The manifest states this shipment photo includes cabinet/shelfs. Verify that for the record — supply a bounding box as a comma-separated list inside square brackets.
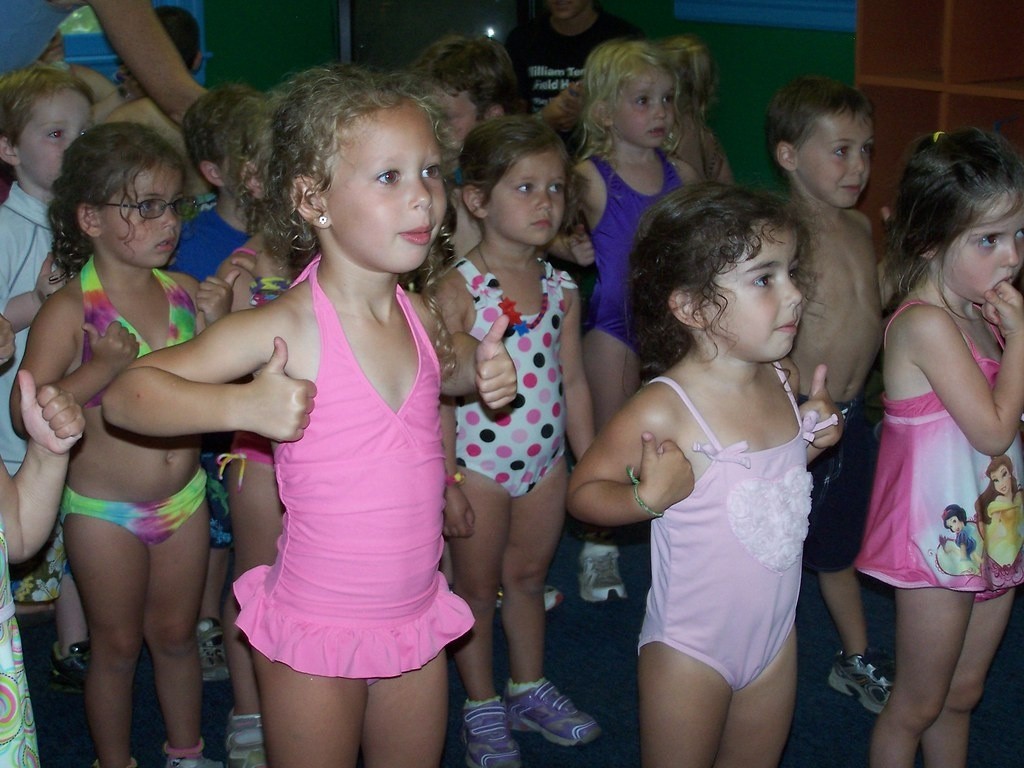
[856, 0, 1024, 263]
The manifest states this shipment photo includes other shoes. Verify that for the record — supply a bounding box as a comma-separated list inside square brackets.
[166, 756, 224, 768]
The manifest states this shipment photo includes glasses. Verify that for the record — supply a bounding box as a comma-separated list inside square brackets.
[85, 195, 195, 220]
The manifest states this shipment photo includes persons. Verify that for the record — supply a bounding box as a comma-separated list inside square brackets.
[1, 0, 1024, 768]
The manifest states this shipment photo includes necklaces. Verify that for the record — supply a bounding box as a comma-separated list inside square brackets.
[476, 242, 549, 336]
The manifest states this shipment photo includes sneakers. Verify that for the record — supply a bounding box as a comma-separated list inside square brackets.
[579, 531, 628, 602]
[502, 677, 601, 747]
[828, 648, 895, 714]
[496, 583, 564, 611]
[224, 705, 268, 768]
[460, 694, 521, 768]
[196, 617, 231, 682]
[38, 637, 92, 699]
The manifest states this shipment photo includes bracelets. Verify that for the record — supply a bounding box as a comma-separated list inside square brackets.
[625, 464, 664, 518]
[248, 292, 279, 307]
[249, 276, 292, 293]
[446, 472, 465, 489]
